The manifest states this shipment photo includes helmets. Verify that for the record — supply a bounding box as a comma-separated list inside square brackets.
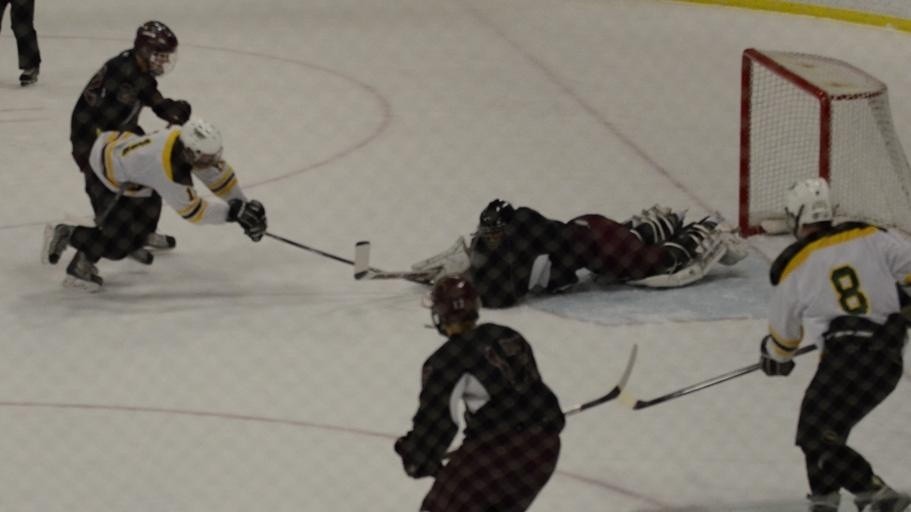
[784, 177, 833, 225]
[429, 272, 478, 335]
[478, 198, 516, 252]
[180, 117, 223, 167]
[137, 21, 178, 74]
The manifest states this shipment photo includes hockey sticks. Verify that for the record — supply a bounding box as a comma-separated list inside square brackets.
[441, 344, 639, 460]
[354, 241, 439, 279]
[615, 341, 818, 410]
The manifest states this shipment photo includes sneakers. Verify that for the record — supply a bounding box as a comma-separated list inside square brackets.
[143, 232, 176, 248]
[49, 224, 78, 264]
[131, 247, 154, 265]
[856, 474, 911, 512]
[66, 249, 103, 287]
[19, 63, 39, 80]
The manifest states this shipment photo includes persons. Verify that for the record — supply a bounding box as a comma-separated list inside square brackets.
[0, 1, 43, 87]
[70, 20, 192, 266]
[41, 118, 267, 294]
[394, 278, 565, 512]
[427, 198, 749, 311]
[757, 176, 910, 512]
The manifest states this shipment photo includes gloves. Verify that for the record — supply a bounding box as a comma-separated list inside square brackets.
[225, 198, 268, 242]
[761, 334, 795, 376]
[154, 99, 192, 125]
[394, 429, 444, 477]
